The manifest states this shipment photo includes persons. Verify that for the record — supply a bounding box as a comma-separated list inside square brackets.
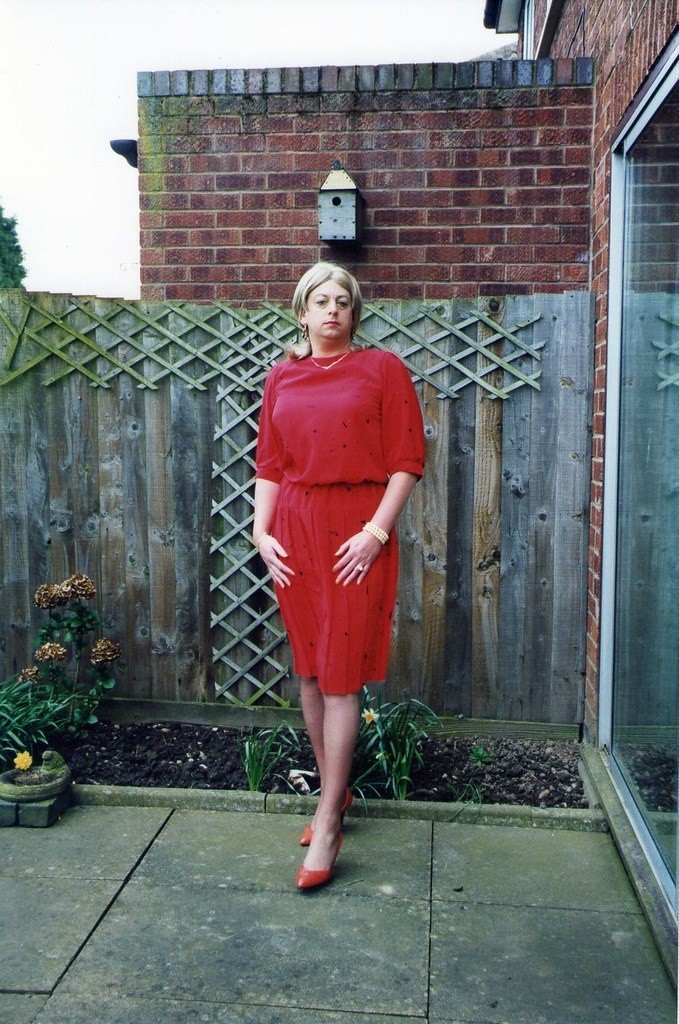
[250, 260, 425, 890]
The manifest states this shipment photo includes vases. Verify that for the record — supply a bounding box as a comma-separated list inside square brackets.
[0, 750, 71, 802]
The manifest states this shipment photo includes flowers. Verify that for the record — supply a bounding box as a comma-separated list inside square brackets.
[14, 750, 32, 770]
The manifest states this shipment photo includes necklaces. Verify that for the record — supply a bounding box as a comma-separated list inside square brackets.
[311, 349, 350, 369]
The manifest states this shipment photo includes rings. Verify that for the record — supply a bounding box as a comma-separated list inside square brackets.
[357, 564, 363, 571]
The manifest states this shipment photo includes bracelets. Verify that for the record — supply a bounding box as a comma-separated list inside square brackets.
[363, 522, 389, 545]
[256, 534, 265, 548]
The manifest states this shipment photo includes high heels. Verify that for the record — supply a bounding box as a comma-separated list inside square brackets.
[298, 784, 353, 846]
[295, 830, 343, 890]
